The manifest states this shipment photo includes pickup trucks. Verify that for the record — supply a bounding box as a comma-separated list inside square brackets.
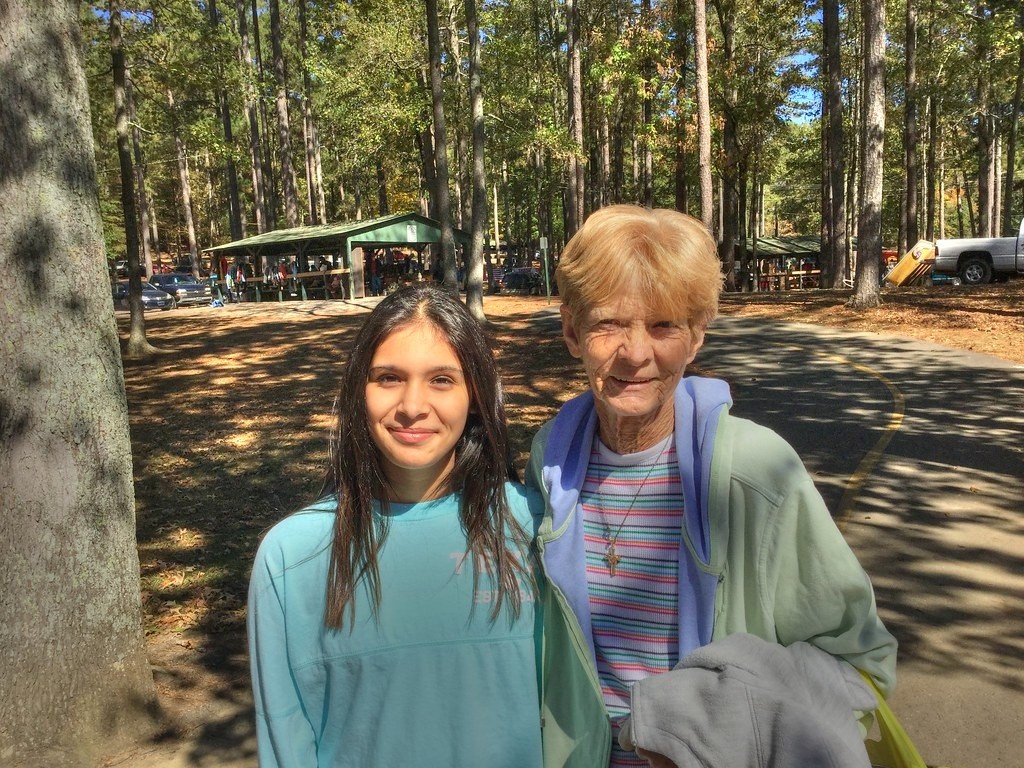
[934, 216, 1024, 286]
[149, 273, 214, 307]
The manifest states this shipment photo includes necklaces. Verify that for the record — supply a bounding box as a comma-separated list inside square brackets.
[597, 418, 676, 576]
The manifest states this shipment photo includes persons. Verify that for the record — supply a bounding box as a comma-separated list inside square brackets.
[365, 253, 386, 296]
[277, 257, 331, 280]
[247, 282, 545, 768]
[525, 204, 897, 768]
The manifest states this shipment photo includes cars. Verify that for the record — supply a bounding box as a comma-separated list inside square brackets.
[114, 259, 129, 276]
[112, 279, 173, 313]
